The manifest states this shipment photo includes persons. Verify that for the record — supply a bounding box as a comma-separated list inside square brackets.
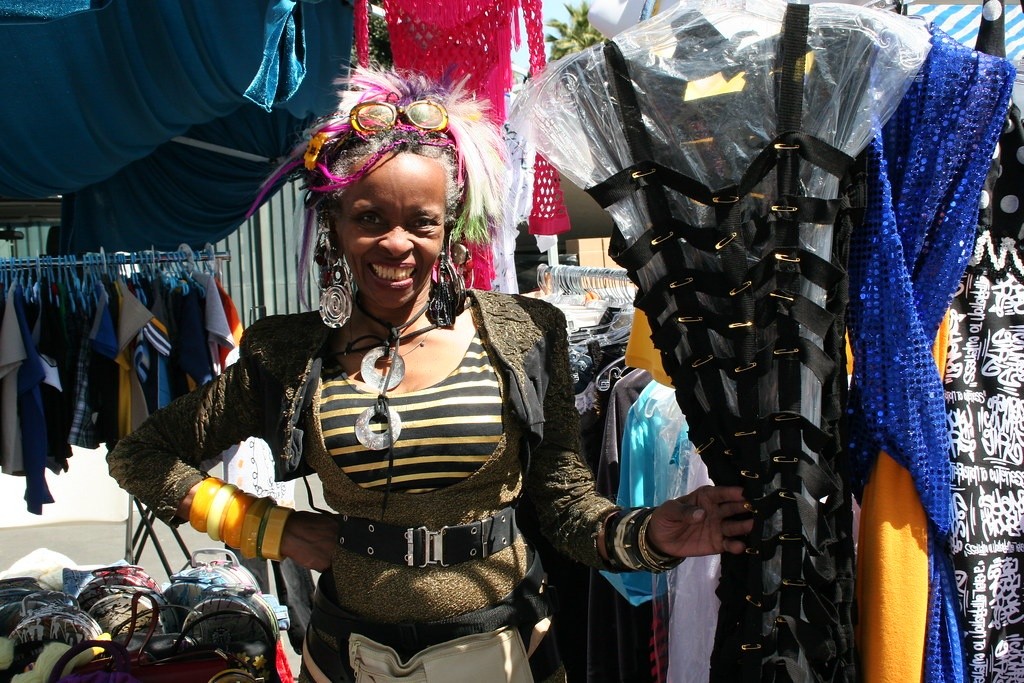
[106, 62, 756, 683]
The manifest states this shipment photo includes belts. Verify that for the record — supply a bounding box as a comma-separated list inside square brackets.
[334, 505, 520, 569]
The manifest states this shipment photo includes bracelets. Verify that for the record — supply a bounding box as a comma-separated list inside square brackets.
[190, 477, 295, 562]
[604, 506, 686, 574]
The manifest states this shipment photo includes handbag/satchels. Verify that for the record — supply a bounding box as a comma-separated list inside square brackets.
[0, 559, 276, 683]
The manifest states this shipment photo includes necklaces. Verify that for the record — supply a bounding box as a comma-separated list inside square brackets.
[352, 300, 434, 451]
[349, 306, 436, 362]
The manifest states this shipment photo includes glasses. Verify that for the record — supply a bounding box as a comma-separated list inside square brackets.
[350, 100, 447, 132]
